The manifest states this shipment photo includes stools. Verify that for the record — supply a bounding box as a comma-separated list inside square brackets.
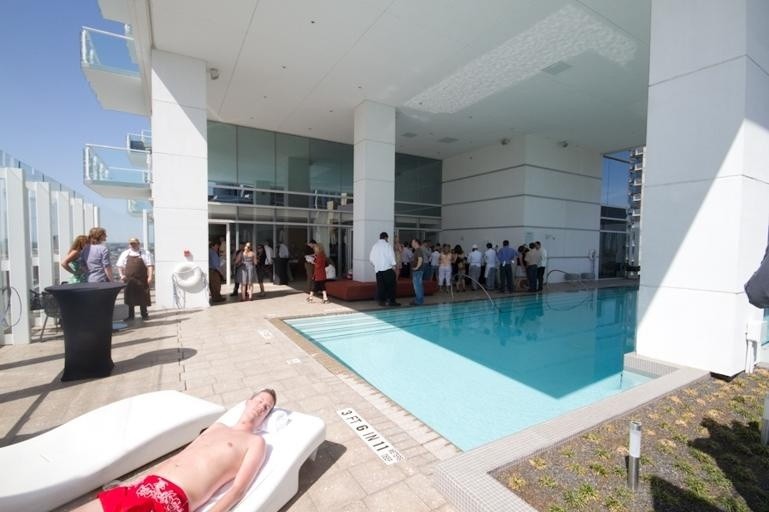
[565, 273, 580, 287]
[581, 272, 595, 285]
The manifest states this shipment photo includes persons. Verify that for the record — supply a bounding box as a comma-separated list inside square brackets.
[61, 235, 88, 284]
[66, 389, 276, 512]
[116, 238, 154, 322]
[81, 228, 113, 283]
[394, 234, 547, 305]
[369, 232, 401, 307]
[209, 235, 336, 304]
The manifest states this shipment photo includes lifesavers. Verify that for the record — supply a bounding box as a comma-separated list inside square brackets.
[174, 263, 202, 289]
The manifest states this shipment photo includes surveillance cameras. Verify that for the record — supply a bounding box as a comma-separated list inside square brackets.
[502, 139, 510, 145]
[562, 142, 568, 148]
[211, 71, 219, 80]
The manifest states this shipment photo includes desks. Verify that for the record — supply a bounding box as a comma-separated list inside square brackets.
[47, 282, 131, 384]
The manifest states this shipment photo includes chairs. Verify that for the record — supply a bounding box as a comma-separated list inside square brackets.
[38, 292, 65, 343]
[0, 389, 231, 512]
[128, 396, 326, 511]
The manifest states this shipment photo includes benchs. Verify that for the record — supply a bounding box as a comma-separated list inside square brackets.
[324, 277, 435, 305]
